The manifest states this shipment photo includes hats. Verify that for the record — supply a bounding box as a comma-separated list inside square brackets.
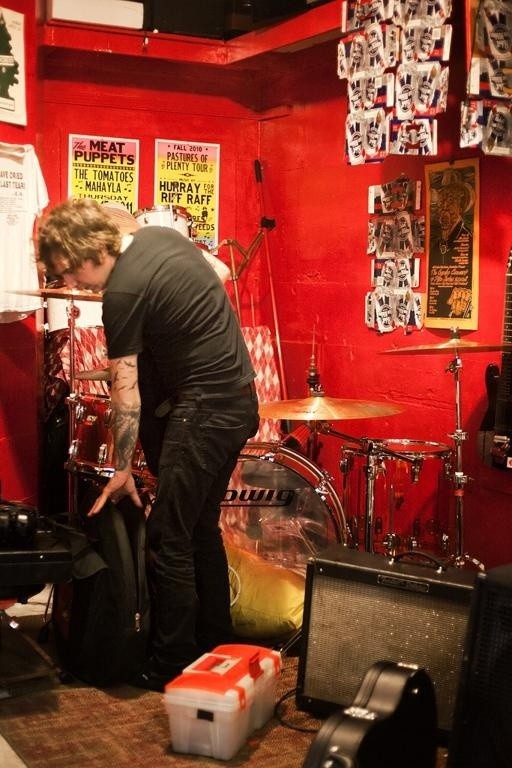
[434, 169, 476, 212]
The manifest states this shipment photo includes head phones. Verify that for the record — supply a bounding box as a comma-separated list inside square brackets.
[0, 498, 41, 546]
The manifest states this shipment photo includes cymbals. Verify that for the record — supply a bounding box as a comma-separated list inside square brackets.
[6, 288, 104, 302]
[69, 366, 109, 380]
[378, 339, 510, 353]
[257, 396, 403, 421]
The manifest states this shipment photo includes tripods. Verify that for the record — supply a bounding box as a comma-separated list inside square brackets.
[432, 359, 487, 574]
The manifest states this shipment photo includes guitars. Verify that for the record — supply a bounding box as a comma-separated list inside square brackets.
[478, 247, 512, 470]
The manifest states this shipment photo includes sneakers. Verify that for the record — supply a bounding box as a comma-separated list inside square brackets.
[138, 661, 181, 686]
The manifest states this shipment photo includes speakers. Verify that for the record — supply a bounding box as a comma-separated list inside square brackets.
[445, 562, 511, 767]
[295, 545, 474, 741]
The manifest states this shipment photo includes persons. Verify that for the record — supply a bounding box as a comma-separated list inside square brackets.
[38, 199, 259, 692]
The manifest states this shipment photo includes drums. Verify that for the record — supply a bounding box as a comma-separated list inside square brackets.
[135, 205, 193, 241]
[340, 439, 451, 564]
[62, 391, 158, 487]
[216, 442, 359, 579]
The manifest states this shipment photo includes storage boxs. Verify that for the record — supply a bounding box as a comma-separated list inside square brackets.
[161, 642, 284, 763]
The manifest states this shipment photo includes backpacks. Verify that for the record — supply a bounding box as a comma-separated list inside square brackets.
[69, 505, 152, 687]
